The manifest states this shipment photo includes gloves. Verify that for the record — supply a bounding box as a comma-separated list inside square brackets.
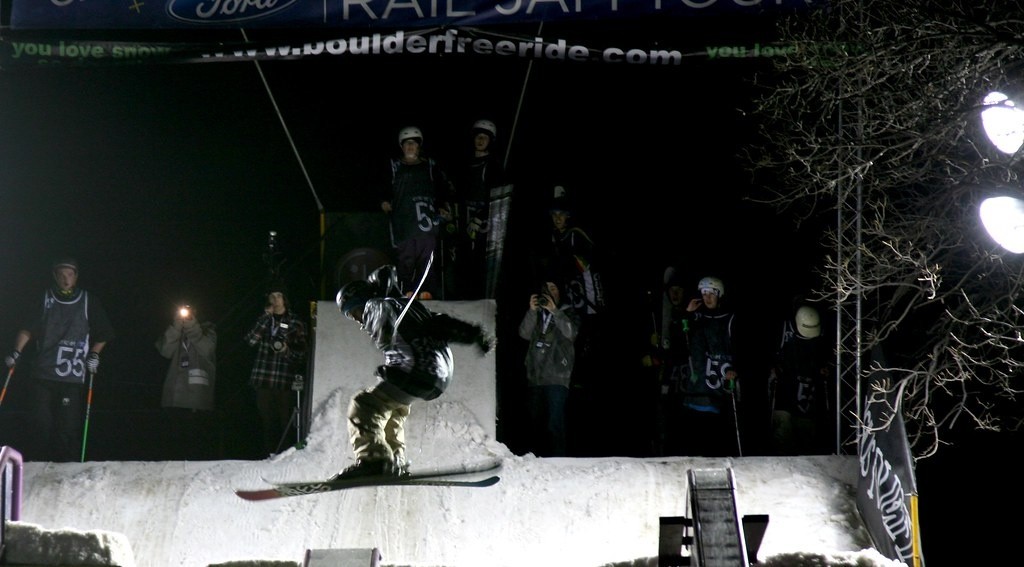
[474, 324, 492, 354]
[4, 349, 22, 368]
[84, 351, 100, 374]
[385, 349, 414, 372]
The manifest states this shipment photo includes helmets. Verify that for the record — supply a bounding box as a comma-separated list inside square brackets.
[398, 126, 424, 149]
[665, 272, 691, 299]
[796, 307, 821, 337]
[336, 280, 374, 317]
[548, 201, 571, 218]
[698, 277, 725, 297]
[50, 257, 82, 277]
[472, 119, 498, 141]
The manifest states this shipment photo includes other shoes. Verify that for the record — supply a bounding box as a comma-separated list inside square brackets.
[406, 291, 419, 299]
[325, 453, 411, 480]
[418, 291, 433, 300]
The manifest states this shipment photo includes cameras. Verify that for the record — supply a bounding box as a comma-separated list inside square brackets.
[268, 323, 288, 352]
[536, 295, 548, 306]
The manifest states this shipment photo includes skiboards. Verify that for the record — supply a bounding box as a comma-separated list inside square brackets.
[235, 460, 505, 502]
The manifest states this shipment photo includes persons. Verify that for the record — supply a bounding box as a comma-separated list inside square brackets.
[328, 280, 494, 478]
[444, 120, 507, 301]
[157, 299, 218, 460]
[371, 127, 458, 301]
[518, 194, 831, 459]
[243, 288, 307, 463]
[5, 256, 115, 466]
